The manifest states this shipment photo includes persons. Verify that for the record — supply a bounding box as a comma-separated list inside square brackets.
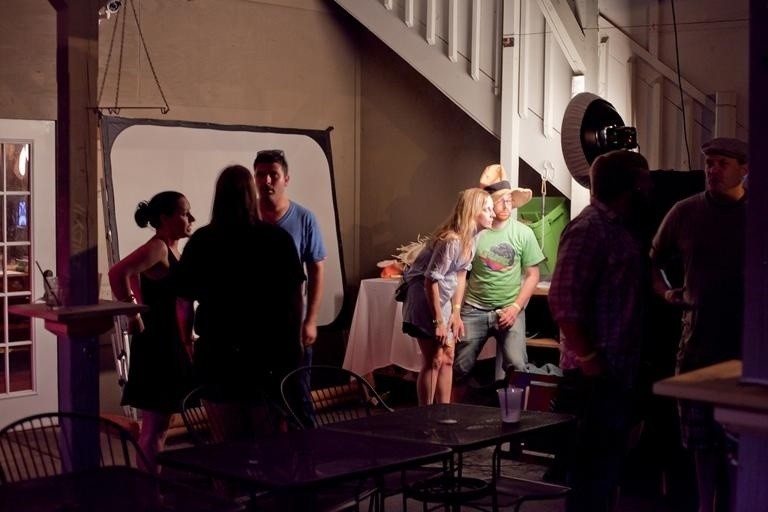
[649, 136, 750, 512]
[395, 188, 497, 405]
[252, 150, 327, 432]
[539, 149, 653, 487]
[108, 190, 196, 473]
[451, 163, 546, 404]
[177, 165, 309, 444]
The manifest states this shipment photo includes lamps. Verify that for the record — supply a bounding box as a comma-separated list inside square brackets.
[559, 92, 639, 193]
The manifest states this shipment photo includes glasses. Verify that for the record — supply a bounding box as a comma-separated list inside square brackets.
[256, 148, 286, 167]
[496, 196, 515, 205]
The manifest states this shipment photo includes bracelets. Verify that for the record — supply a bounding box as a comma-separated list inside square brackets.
[120, 294, 136, 303]
[511, 302, 522, 312]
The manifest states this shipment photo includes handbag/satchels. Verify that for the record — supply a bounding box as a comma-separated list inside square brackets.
[393, 273, 426, 304]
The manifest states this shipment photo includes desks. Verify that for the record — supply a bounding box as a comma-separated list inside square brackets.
[1, 464, 240, 512]
[322, 402, 574, 512]
[156, 431, 454, 512]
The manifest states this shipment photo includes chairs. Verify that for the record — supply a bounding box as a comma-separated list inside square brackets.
[180, 377, 379, 512]
[1, 414, 152, 483]
[280, 365, 452, 512]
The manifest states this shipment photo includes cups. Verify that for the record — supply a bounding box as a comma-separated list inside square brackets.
[497, 388, 523, 429]
[43, 277, 67, 311]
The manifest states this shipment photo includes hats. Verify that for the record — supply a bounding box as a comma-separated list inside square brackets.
[479, 161, 532, 213]
[700, 137, 749, 164]
